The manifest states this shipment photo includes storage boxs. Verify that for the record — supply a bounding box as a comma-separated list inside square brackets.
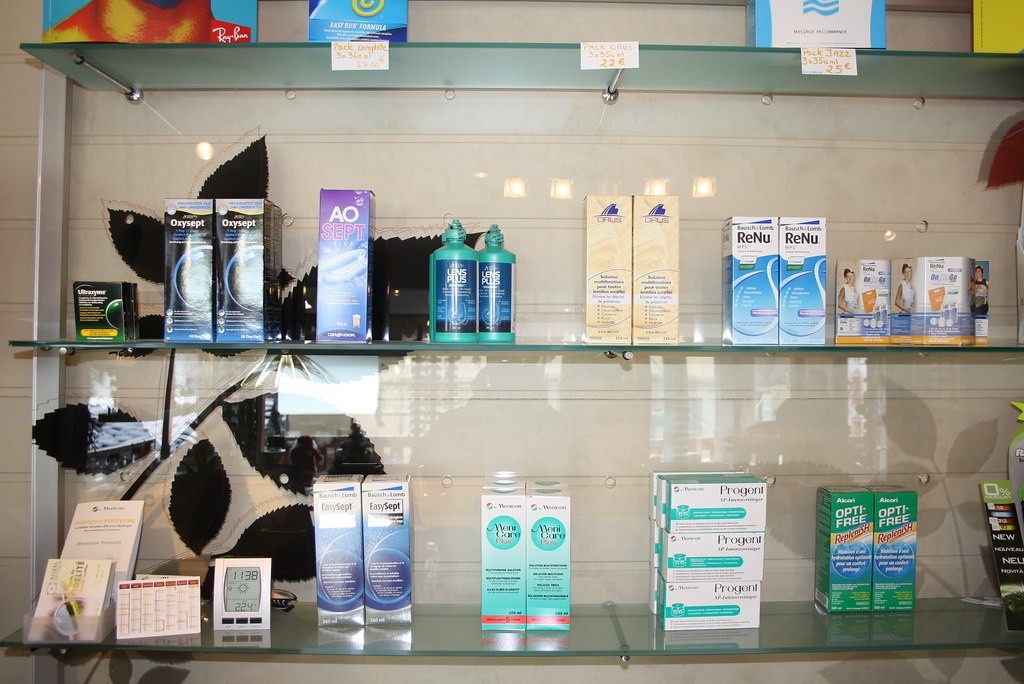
[583, 196, 680, 345]
[744, 0, 885, 49]
[482, 480, 572, 633]
[649, 469, 767, 631]
[74, 282, 140, 342]
[815, 484, 917, 613]
[720, 216, 826, 346]
[166, 197, 284, 345]
[316, 187, 375, 343]
[313, 473, 413, 627]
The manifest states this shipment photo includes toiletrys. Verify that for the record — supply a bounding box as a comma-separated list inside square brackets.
[425, 217, 481, 348]
[478, 222, 519, 345]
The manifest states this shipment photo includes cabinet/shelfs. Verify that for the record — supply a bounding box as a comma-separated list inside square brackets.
[19, 42, 1024, 684]
